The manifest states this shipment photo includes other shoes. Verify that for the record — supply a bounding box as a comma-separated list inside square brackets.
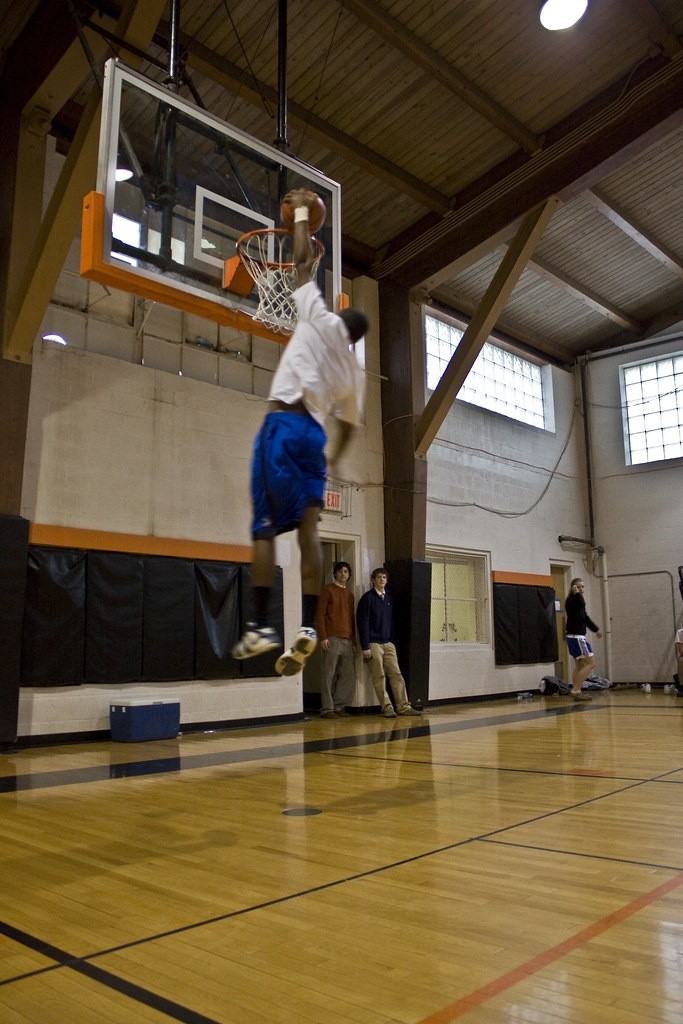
[640, 683, 651, 693]
[401, 708, 422, 716]
[325, 711, 339, 719]
[677, 687, 683, 697]
[573, 692, 591, 701]
[336, 709, 349, 718]
[384, 708, 396, 718]
[663, 684, 674, 694]
[569, 691, 587, 700]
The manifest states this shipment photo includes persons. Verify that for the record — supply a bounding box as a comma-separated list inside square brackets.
[675, 620, 683, 697]
[317, 559, 358, 719]
[562, 578, 603, 701]
[231, 189, 372, 677]
[356, 568, 420, 718]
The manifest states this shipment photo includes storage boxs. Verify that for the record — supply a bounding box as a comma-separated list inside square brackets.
[109, 696, 180, 742]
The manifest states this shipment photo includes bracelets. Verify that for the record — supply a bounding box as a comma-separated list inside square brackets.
[293, 204, 310, 224]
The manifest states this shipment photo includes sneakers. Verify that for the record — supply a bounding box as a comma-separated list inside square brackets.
[231, 621, 281, 660]
[276, 627, 316, 676]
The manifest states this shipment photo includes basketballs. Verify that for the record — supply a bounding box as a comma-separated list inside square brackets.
[280, 188, 327, 235]
